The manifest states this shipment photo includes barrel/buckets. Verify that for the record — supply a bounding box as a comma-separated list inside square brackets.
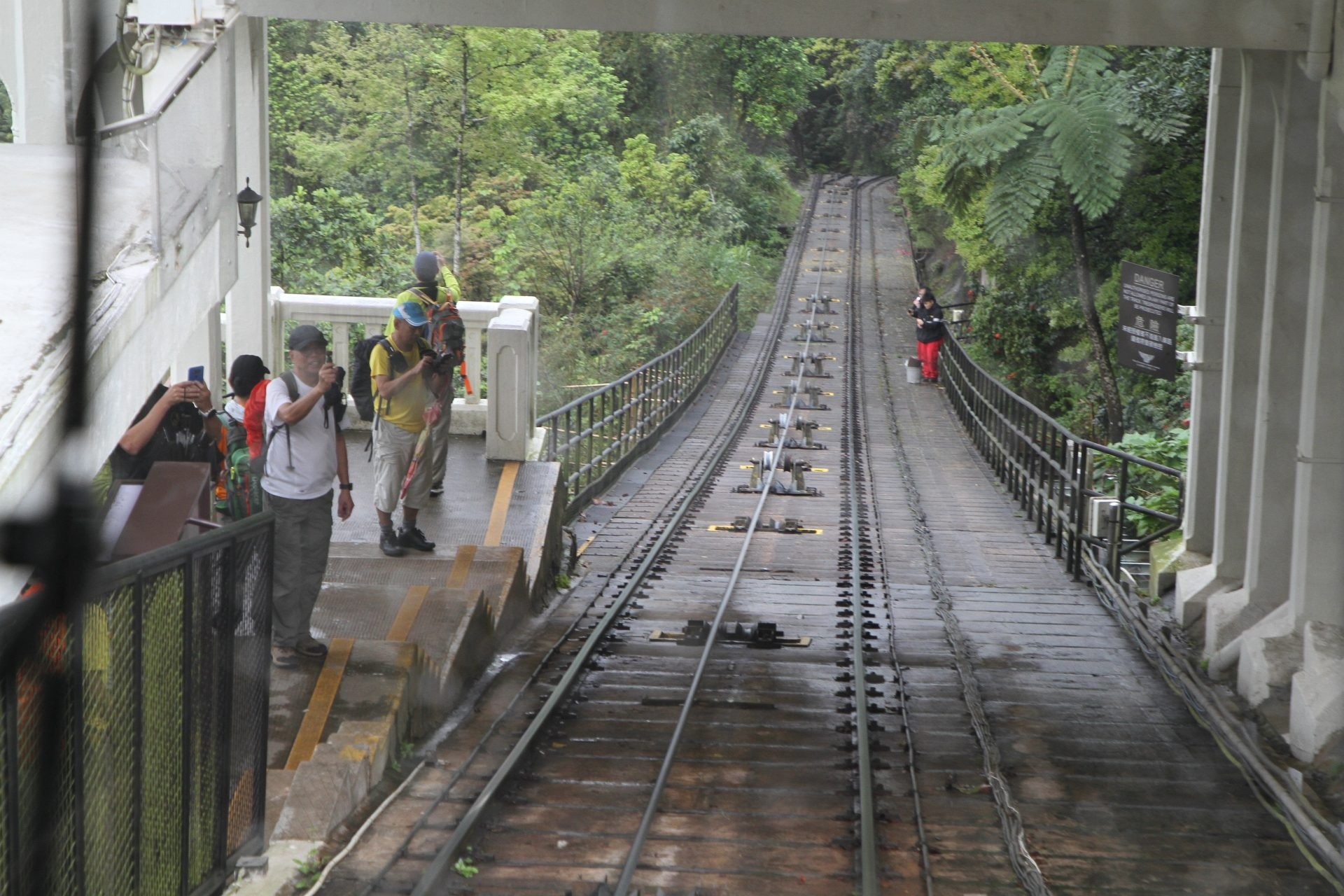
[906, 366, 922, 383]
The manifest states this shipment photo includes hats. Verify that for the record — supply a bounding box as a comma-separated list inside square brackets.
[289, 324, 328, 351]
[918, 292, 933, 303]
[230, 355, 271, 377]
[394, 302, 432, 326]
[414, 251, 438, 276]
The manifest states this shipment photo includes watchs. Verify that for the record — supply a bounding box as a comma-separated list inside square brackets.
[197, 408, 215, 420]
[340, 482, 353, 491]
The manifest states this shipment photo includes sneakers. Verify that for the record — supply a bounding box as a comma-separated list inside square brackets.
[380, 530, 408, 557]
[398, 527, 436, 550]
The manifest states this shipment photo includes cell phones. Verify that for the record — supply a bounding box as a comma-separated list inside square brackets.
[187, 366, 204, 381]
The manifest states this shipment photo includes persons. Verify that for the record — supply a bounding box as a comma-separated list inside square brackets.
[908, 286, 944, 384]
[384, 249, 461, 496]
[213, 353, 273, 637]
[259, 324, 356, 669]
[109, 378, 225, 482]
[369, 302, 452, 556]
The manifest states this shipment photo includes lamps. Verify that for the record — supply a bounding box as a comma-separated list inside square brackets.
[237, 177, 263, 247]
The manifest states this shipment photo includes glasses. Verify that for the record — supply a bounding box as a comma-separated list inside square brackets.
[296, 344, 325, 358]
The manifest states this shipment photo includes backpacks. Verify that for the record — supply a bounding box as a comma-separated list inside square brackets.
[213, 410, 265, 520]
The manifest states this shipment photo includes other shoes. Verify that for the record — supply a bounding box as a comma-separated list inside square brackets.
[270, 645, 299, 667]
[295, 636, 328, 655]
[211, 612, 242, 629]
[430, 485, 444, 496]
[254, 621, 272, 638]
[923, 378, 937, 385]
